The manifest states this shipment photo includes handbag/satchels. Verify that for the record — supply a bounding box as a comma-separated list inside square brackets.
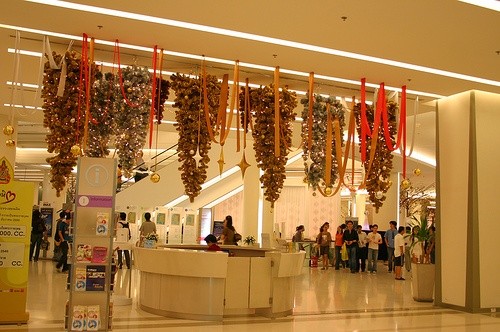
[233, 233, 241, 242]
[328, 248, 333, 259]
[342, 243, 348, 260]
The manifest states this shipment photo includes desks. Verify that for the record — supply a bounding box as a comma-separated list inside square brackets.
[296, 241, 335, 266]
[112, 241, 135, 305]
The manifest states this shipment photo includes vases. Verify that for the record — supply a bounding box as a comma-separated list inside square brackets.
[148, 239, 157, 246]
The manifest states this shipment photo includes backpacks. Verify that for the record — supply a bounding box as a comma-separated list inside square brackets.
[118, 222, 131, 240]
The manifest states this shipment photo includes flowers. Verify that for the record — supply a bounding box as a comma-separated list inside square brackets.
[146, 230, 159, 241]
[244, 235, 256, 246]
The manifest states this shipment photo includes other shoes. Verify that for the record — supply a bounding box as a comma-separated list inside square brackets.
[320, 268, 325, 270]
[361, 269, 365, 272]
[395, 277, 406, 280]
[119, 263, 123, 269]
[367, 271, 377, 274]
[56, 268, 62, 273]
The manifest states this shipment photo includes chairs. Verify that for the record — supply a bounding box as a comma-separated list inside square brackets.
[279, 238, 346, 268]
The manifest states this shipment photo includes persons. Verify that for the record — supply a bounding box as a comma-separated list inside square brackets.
[53, 211, 71, 274]
[139, 212, 157, 242]
[29, 210, 46, 263]
[115, 212, 131, 270]
[316, 221, 382, 274]
[204, 215, 236, 251]
[293, 225, 310, 250]
[384, 221, 435, 280]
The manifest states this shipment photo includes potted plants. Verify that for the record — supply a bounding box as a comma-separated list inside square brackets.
[403, 213, 436, 303]
